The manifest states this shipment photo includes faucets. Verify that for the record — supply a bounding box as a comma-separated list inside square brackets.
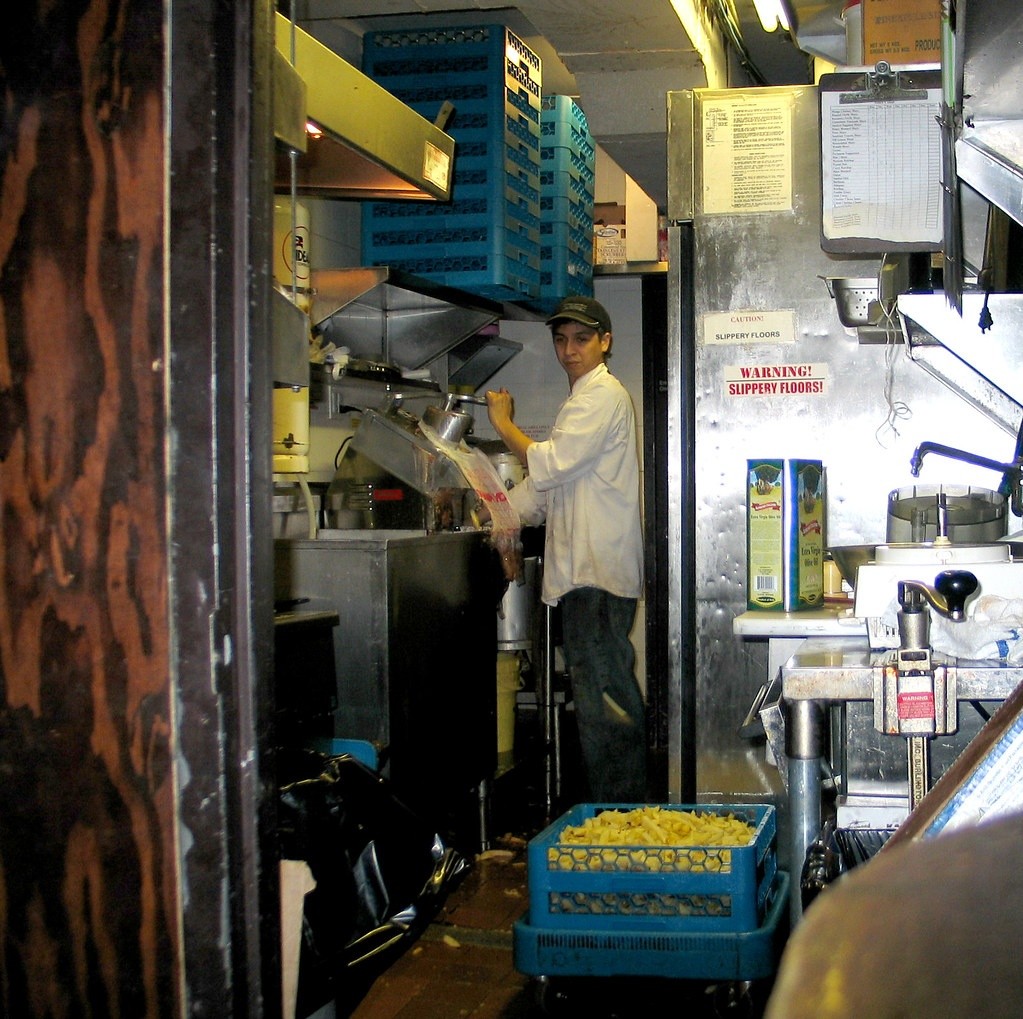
[910, 441, 1021, 479]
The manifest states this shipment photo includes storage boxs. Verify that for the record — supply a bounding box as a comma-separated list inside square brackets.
[357, 20, 597, 310]
[513, 797, 790, 981]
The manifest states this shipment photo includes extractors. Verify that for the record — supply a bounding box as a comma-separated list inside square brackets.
[311, 267, 503, 370]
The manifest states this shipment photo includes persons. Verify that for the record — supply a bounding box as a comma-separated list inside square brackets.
[487, 298, 647, 801]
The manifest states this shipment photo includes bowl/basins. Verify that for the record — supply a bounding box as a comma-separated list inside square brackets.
[825, 545, 875, 589]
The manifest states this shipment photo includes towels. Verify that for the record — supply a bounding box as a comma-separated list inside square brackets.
[880, 593, 1022, 659]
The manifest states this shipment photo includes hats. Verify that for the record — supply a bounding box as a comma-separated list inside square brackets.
[545, 296, 611, 331]
